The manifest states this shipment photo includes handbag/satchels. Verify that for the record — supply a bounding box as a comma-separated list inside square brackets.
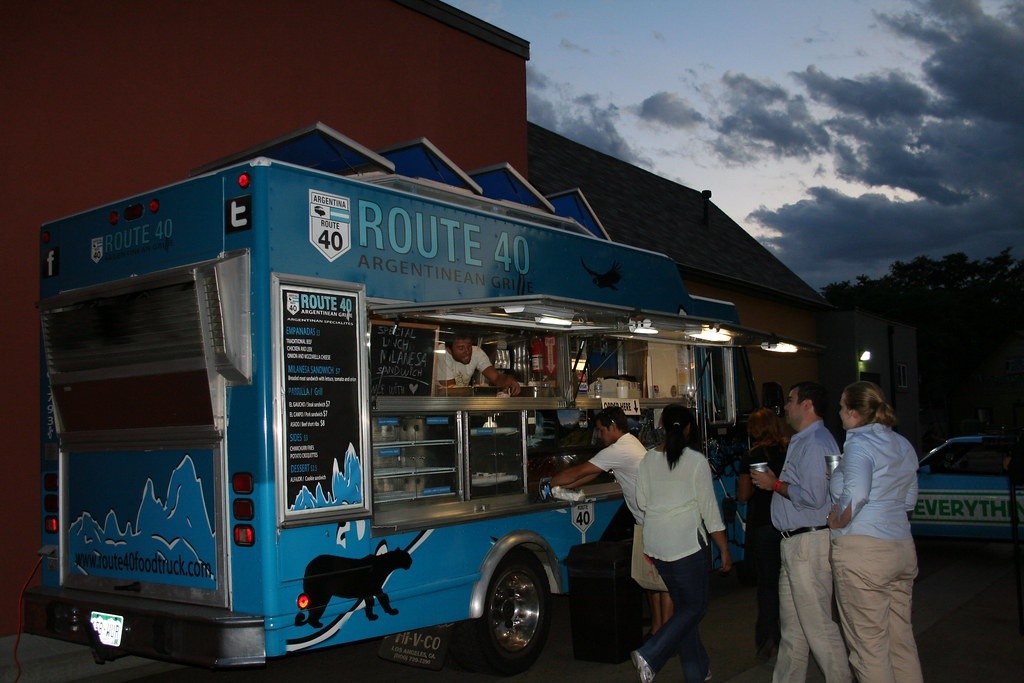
[629, 521, 670, 593]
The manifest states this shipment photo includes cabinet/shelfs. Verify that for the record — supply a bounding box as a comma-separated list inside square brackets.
[371, 408, 528, 505]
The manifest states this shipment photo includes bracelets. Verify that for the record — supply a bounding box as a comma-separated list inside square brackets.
[773, 479, 782, 491]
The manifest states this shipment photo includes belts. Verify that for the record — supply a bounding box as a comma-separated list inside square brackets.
[781, 524, 829, 540]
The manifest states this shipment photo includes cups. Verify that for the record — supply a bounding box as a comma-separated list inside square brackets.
[617, 380, 641, 399]
[825, 455, 841, 479]
[749, 463, 768, 473]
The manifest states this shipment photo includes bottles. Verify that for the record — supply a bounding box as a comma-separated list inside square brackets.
[594, 378, 603, 398]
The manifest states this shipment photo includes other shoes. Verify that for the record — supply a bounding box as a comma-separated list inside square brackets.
[630, 648, 658, 683]
[704, 668, 713, 681]
[756, 634, 775, 663]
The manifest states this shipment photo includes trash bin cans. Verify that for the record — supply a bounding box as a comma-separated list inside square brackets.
[560, 540, 643, 663]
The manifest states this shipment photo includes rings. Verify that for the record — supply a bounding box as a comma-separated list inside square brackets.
[754, 481, 757, 484]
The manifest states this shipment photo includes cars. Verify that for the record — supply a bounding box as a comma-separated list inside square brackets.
[906, 433, 1024, 553]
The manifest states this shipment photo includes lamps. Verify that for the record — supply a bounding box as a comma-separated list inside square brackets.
[762, 341, 798, 354]
[683, 326, 732, 342]
[507, 305, 575, 326]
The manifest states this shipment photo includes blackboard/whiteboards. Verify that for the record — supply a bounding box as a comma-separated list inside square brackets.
[367, 319, 439, 397]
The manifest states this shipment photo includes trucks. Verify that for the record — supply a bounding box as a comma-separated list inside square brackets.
[19, 158, 788, 678]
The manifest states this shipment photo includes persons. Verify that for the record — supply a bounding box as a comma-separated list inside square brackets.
[630, 404, 733, 683]
[550, 406, 673, 638]
[750, 381, 855, 683]
[738, 408, 788, 667]
[434, 326, 521, 396]
[827, 381, 923, 683]
